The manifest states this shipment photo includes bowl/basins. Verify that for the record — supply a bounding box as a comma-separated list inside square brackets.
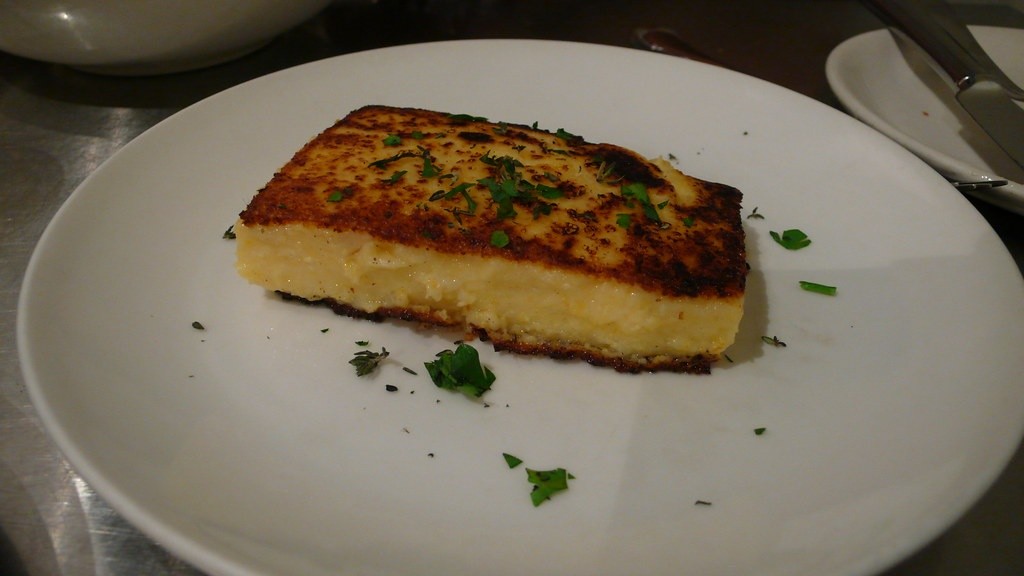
[0, 0, 336, 76]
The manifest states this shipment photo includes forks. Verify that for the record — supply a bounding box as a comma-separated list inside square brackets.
[636, 29, 1008, 192]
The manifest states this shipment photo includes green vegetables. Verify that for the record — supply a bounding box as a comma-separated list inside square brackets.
[327, 117, 838, 502]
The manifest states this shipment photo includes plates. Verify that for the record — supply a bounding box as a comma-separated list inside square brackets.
[18, 39, 1024, 576]
[825, 22, 1024, 211]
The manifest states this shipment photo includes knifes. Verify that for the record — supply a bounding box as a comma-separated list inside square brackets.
[866, 0, 1024, 170]
